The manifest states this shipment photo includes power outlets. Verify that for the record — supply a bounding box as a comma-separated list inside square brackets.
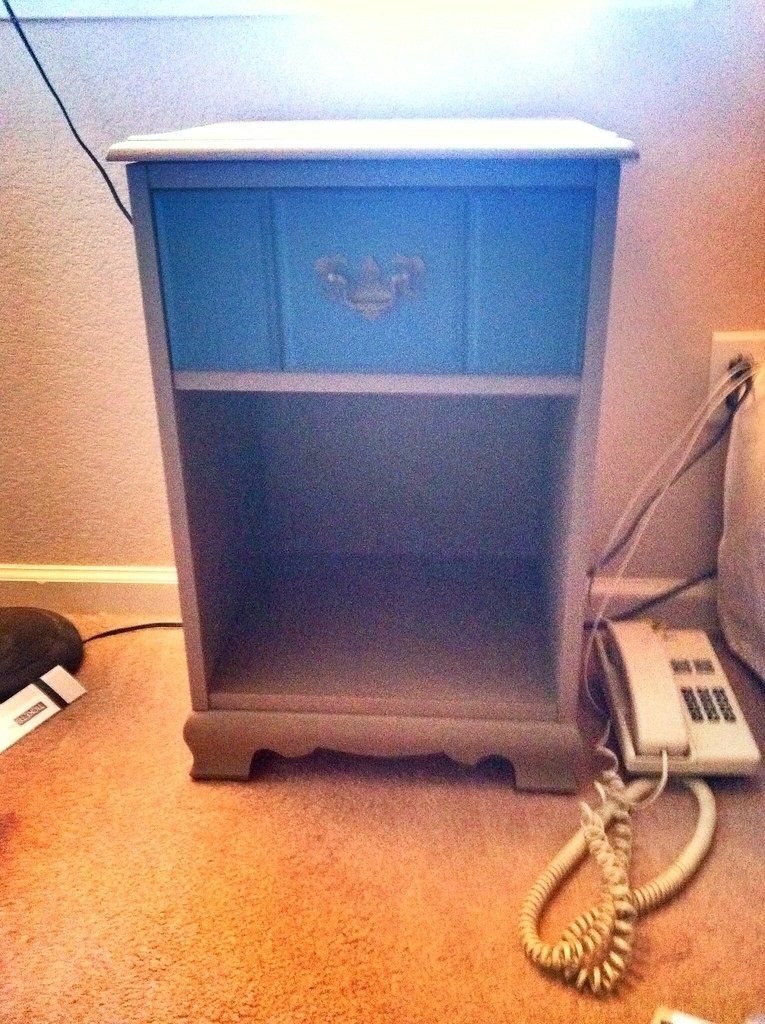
[705, 330, 765, 422]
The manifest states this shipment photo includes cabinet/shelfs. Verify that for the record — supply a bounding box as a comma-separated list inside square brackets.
[105, 120, 643, 800]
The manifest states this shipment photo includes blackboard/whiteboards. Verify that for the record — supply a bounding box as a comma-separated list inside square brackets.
[593, 622, 761, 775]
